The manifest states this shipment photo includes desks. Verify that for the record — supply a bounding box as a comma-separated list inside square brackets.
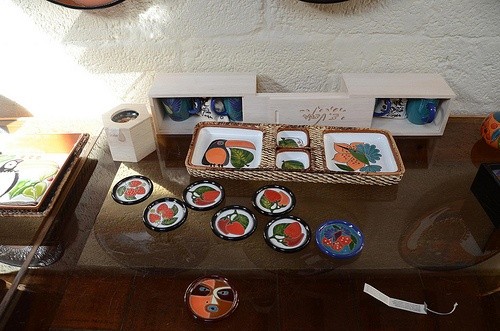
[0, 116, 500, 330]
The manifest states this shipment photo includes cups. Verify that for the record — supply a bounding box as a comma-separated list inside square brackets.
[163, 98, 200, 122]
[211, 97, 243, 122]
[406, 98, 441, 125]
